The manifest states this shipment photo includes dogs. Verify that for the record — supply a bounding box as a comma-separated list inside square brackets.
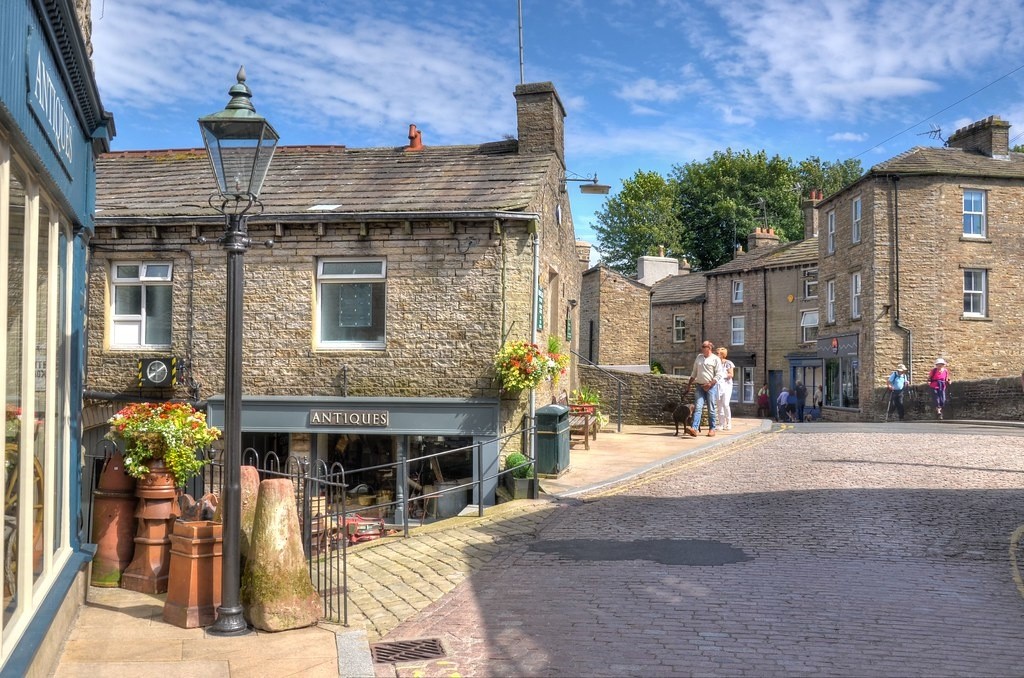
[672, 403, 701, 436]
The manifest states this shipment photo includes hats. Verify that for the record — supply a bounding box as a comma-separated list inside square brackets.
[896, 364, 908, 372]
[934, 359, 946, 365]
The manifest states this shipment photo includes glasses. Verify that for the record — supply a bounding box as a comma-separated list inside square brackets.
[702, 346, 709, 348]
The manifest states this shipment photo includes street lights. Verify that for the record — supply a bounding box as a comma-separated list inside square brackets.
[195, 65, 280, 639]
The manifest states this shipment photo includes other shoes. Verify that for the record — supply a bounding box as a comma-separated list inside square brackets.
[726, 423, 731, 430]
[899, 417, 905, 421]
[718, 425, 724, 431]
[938, 413, 943, 419]
[708, 429, 715, 436]
[686, 428, 698, 437]
[888, 413, 897, 419]
[937, 408, 941, 415]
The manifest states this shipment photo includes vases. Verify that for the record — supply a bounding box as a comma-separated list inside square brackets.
[130, 430, 169, 461]
[500, 391, 519, 399]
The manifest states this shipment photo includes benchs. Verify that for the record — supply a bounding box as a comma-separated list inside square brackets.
[552, 389, 604, 450]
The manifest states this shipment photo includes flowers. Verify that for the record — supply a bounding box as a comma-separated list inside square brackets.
[103, 400, 222, 489]
[493, 339, 550, 399]
[543, 332, 571, 389]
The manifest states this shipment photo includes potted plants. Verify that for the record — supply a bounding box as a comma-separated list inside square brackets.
[566, 385, 608, 435]
[504, 451, 540, 499]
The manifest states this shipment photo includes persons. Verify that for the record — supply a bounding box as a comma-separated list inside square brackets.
[927, 359, 952, 420]
[685, 340, 722, 437]
[776, 380, 823, 423]
[757, 383, 771, 418]
[714, 346, 735, 431]
[886, 363, 910, 421]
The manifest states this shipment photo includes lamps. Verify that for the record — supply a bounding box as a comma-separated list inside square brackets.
[559, 170, 611, 194]
[196, 64, 281, 216]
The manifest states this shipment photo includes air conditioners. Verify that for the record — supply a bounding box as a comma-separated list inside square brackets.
[139, 356, 183, 388]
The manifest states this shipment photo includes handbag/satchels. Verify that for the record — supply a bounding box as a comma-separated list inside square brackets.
[818, 401, 822, 406]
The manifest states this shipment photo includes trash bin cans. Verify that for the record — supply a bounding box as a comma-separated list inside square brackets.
[535, 404, 570, 473]
